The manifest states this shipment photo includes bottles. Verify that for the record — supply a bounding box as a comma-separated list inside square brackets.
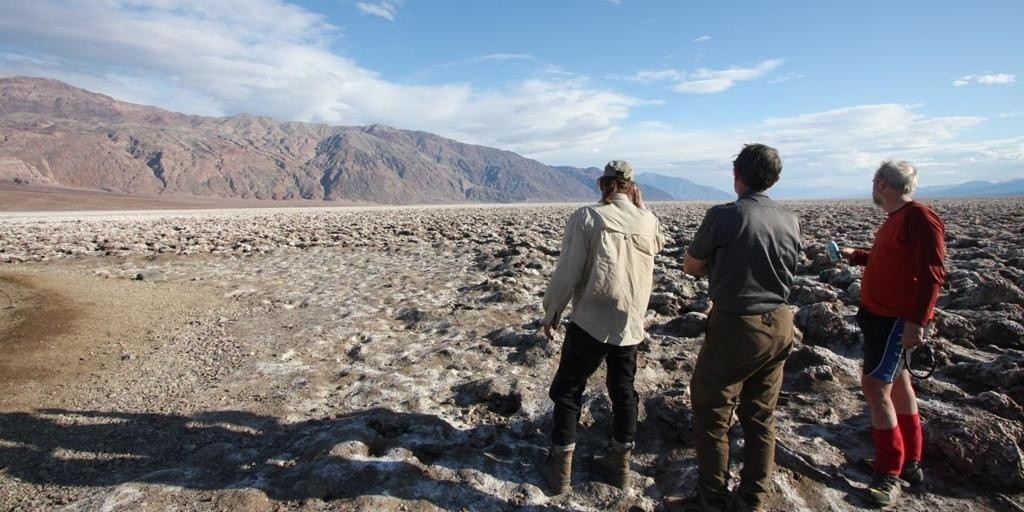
[825, 236, 842, 264]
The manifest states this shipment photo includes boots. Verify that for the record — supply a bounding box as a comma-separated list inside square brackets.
[536, 442, 576, 494]
[581, 437, 635, 488]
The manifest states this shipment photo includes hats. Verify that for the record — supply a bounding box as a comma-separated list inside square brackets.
[604, 160, 634, 182]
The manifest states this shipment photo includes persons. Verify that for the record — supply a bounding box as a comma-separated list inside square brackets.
[825, 160, 946, 508]
[534, 158, 665, 495]
[662, 143, 802, 511]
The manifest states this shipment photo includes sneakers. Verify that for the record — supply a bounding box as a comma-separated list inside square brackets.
[864, 468, 923, 504]
[663, 485, 762, 512]
